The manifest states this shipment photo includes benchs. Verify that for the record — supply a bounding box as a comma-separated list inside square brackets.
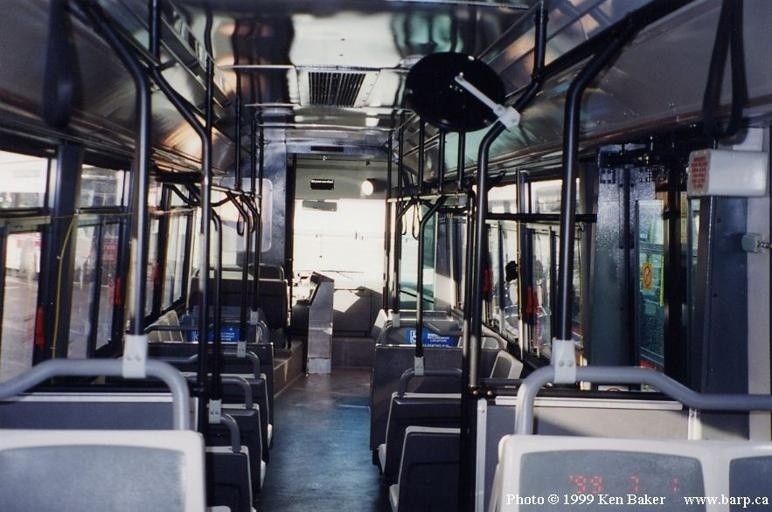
[0, 262, 291, 512]
[367, 317, 772, 511]
